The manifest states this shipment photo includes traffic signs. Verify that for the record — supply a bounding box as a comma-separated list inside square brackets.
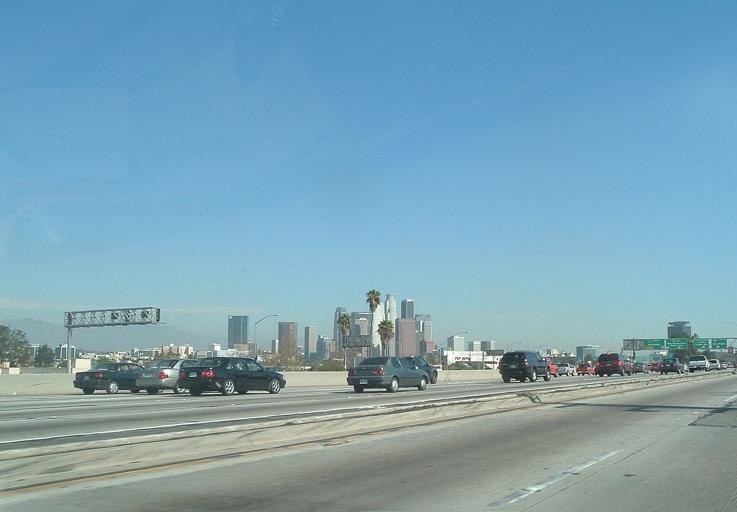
[643, 339, 665, 349]
[666, 338, 688, 350]
[712, 338, 728, 349]
[693, 338, 709, 349]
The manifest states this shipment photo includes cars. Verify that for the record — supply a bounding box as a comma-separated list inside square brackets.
[496, 350, 734, 382]
[346, 355, 439, 392]
[72, 356, 287, 396]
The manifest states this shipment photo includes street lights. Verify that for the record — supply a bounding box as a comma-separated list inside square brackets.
[255, 313, 279, 363]
